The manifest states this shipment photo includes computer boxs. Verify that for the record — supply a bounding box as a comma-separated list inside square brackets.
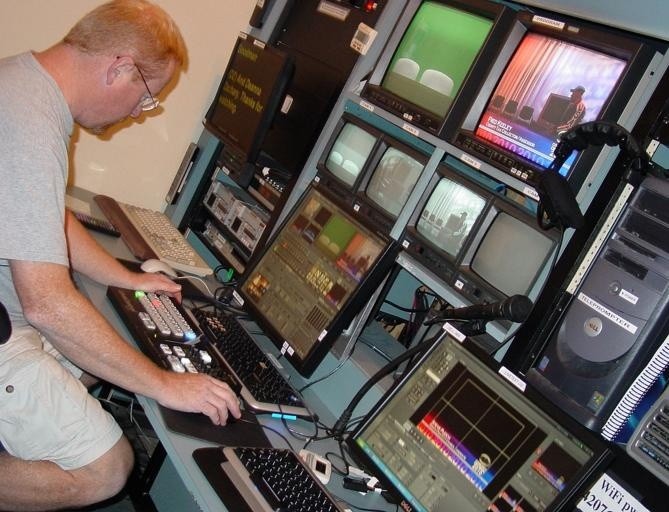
[522, 171, 669, 434]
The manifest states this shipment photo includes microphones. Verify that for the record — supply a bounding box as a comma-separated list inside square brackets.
[431, 294, 534, 324]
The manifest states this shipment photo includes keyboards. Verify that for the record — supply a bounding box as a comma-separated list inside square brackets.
[186, 307, 317, 420]
[219, 445, 344, 511]
[120, 201, 215, 278]
[105, 284, 243, 398]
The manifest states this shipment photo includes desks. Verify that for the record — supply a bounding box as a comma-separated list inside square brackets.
[58, 183, 408, 512]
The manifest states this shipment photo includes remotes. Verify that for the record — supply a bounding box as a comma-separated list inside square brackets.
[72, 212, 121, 236]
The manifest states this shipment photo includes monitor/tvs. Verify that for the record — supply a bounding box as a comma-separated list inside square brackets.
[204, 32, 292, 168]
[355, 135, 431, 223]
[407, 165, 495, 270]
[459, 198, 564, 299]
[235, 182, 403, 378]
[316, 111, 385, 195]
[450, 10, 650, 205]
[360, 0, 514, 142]
[347, 323, 615, 511]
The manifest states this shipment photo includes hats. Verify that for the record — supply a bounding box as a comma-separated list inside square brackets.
[570, 85, 585, 92]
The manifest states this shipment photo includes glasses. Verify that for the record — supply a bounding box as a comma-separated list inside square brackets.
[117, 56, 160, 112]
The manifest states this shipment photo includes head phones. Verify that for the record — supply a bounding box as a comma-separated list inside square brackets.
[531, 117, 649, 233]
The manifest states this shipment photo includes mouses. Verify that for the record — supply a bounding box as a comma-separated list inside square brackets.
[140, 258, 178, 281]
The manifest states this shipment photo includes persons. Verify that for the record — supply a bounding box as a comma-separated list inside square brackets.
[1, 1, 241, 511]
[542, 86, 586, 138]
